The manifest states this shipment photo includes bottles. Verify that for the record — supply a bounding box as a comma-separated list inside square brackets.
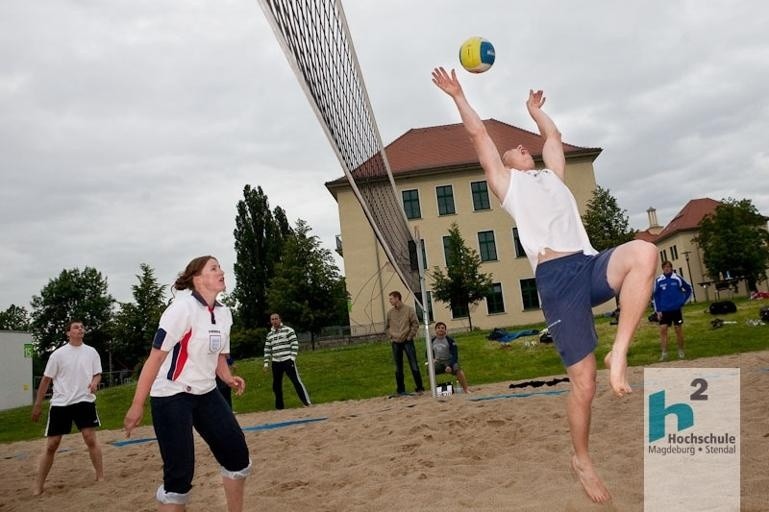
[455, 380, 462, 394]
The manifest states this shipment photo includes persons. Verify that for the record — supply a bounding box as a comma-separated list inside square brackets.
[123, 255, 253, 512]
[424, 322, 472, 394]
[650, 261, 694, 362]
[30, 319, 104, 497]
[384, 291, 425, 399]
[263, 313, 311, 410]
[429, 63, 659, 506]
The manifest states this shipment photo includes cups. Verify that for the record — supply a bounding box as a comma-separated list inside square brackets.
[446, 384, 452, 395]
[436, 385, 442, 398]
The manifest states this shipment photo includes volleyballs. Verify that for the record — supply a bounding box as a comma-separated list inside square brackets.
[459, 36, 495, 72]
